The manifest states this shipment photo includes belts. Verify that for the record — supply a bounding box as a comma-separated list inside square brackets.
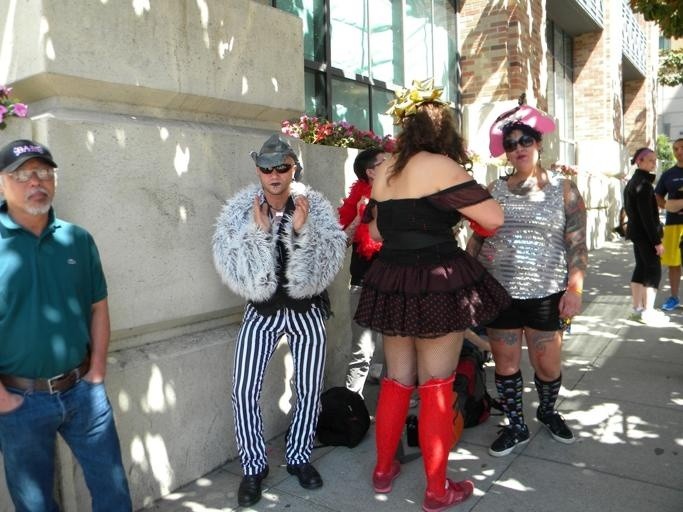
[1, 359, 93, 394]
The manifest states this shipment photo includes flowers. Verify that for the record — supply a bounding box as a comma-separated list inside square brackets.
[385, 78, 444, 125]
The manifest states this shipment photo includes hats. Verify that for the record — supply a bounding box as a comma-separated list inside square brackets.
[0, 140, 57, 173]
[490, 105, 557, 158]
[250, 134, 299, 169]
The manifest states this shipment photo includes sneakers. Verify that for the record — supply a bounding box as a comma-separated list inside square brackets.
[373, 459, 401, 493]
[630, 308, 671, 324]
[662, 296, 681, 311]
[421, 477, 474, 512]
[536, 405, 576, 444]
[489, 424, 530, 457]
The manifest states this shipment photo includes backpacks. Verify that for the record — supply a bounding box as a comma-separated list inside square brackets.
[315, 387, 371, 449]
[453, 338, 504, 428]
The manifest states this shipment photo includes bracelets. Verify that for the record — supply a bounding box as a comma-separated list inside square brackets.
[470, 223, 496, 238]
[569, 288, 586, 295]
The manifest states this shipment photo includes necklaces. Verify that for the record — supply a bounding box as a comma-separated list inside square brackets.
[267, 202, 285, 217]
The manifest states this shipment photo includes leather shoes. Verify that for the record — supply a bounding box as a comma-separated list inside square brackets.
[238, 465, 269, 507]
[287, 463, 323, 489]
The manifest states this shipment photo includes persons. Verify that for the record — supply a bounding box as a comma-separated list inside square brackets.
[212, 132, 350, 506]
[654, 138, 683, 311]
[466, 92, 589, 458]
[623, 147, 671, 322]
[337, 147, 392, 399]
[352, 80, 504, 512]
[0, 139, 134, 511]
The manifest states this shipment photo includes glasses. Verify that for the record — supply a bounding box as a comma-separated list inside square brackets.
[259, 164, 291, 174]
[504, 134, 535, 152]
[8, 167, 54, 182]
